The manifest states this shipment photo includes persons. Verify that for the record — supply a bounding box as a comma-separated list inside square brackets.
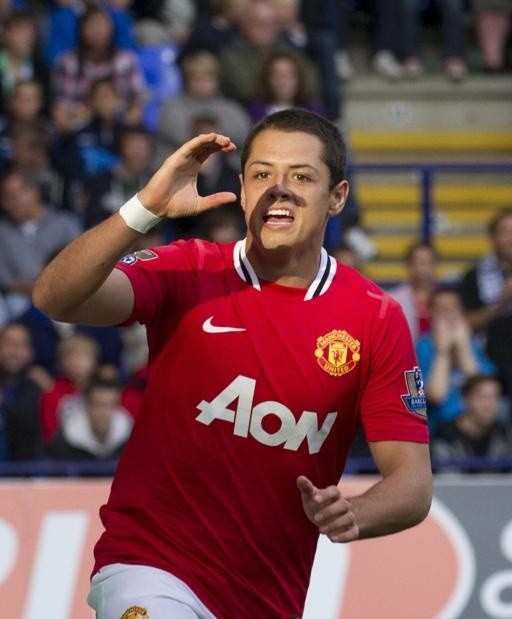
[1, 1, 512, 475]
[31, 104, 435, 619]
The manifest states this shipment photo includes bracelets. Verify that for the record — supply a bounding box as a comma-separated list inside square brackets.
[119, 191, 162, 235]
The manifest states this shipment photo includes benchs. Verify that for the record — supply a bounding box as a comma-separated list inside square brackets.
[339, 145, 510, 295]
